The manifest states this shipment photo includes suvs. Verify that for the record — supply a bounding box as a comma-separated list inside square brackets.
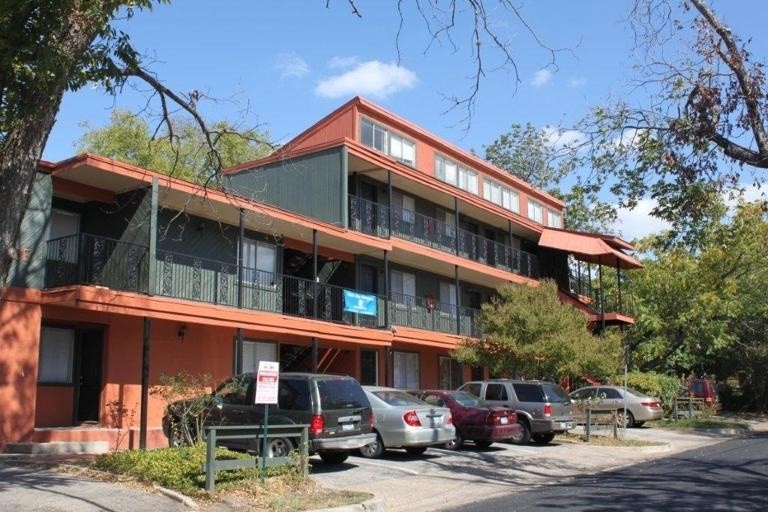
[457, 377, 576, 446]
[162, 371, 377, 465]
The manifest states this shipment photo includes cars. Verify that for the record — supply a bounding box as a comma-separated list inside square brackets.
[361, 384, 457, 459]
[676, 377, 721, 417]
[406, 390, 523, 450]
[568, 385, 664, 428]
